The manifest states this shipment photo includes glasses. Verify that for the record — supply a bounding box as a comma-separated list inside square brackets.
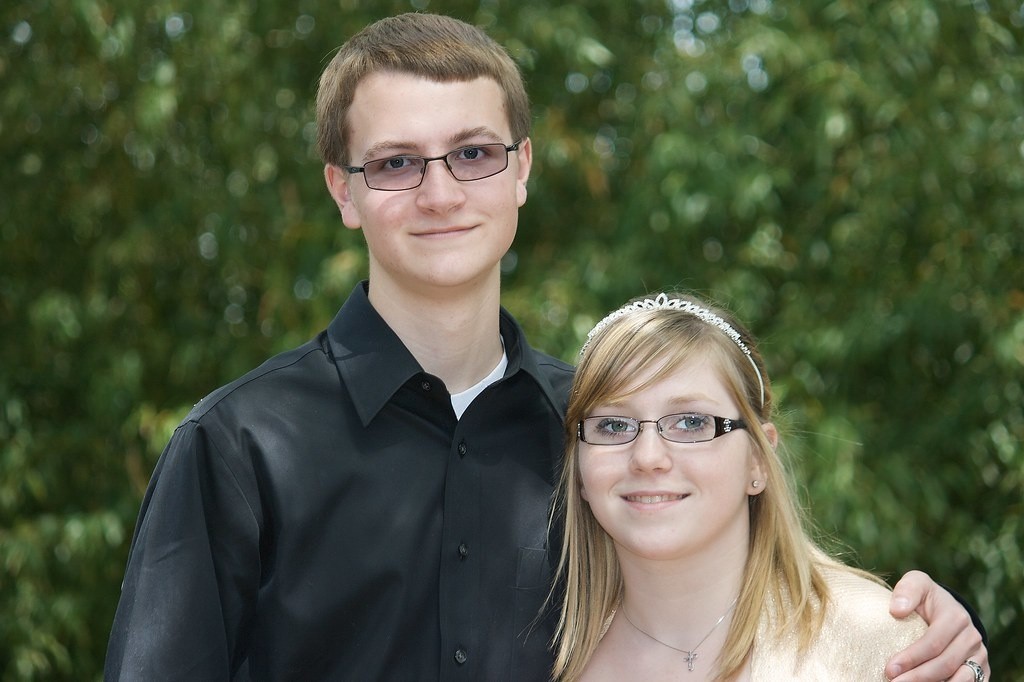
[576, 412, 745, 446]
[347, 141, 520, 191]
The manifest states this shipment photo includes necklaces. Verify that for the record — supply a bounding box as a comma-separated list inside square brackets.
[612, 598, 735, 672]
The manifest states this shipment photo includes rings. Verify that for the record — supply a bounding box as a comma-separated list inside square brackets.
[961, 658, 985, 682]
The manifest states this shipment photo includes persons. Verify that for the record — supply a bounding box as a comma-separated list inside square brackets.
[95, 15, 993, 682]
[530, 289, 934, 682]
